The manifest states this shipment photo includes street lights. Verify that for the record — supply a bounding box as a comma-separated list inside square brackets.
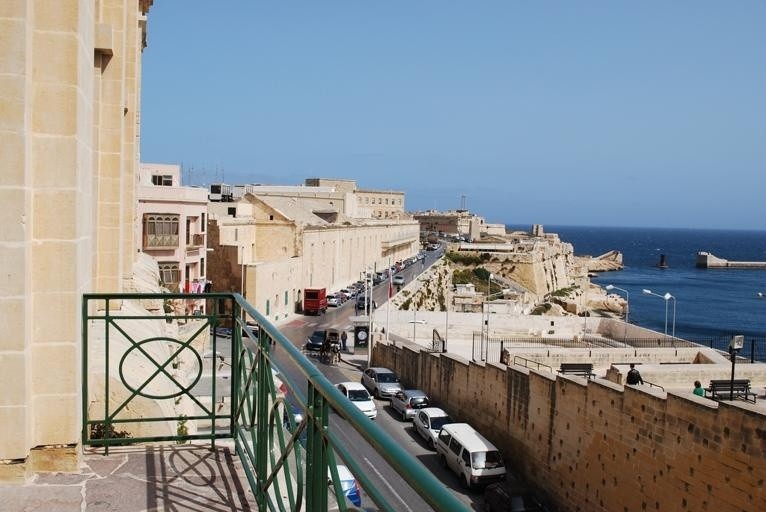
[408, 319, 430, 341]
[665, 291, 678, 338]
[606, 284, 630, 347]
[643, 288, 668, 338]
[728, 333, 745, 403]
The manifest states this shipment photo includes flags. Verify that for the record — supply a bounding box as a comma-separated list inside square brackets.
[386, 263, 395, 301]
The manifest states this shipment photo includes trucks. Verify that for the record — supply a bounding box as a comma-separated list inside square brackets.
[302, 286, 328, 317]
[209, 184, 232, 201]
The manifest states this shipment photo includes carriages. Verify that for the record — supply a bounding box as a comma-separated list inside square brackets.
[319, 327, 342, 366]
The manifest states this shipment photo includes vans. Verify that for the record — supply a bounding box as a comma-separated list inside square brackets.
[436, 421, 509, 490]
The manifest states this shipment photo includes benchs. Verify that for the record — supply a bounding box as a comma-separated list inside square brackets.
[702, 378, 749, 401]
[556, 363, 597, 381]
[711, 380, 758, 404]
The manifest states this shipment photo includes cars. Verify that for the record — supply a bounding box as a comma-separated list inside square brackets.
[412, 407, 455, 450]
[326, 463, 362, 507]
[389, 388, 432, 422]
[324, 250, 427, 309]
[306, 329, 325, 351]
[360, 366, 405, 400]
[277, 401, 308, 449]
[482, 479, 551, 512]
[331, 379, 378, 420]
[419, 231, 466, 252]
[245, 319, 259, 334]
[270, 368, 288, 400]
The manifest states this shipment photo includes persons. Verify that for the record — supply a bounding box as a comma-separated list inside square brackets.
[692, 380, 704, 397]
[421, 255, 425, 272]
[625, 362, 644, 386]
[340, 330, 348, 351]
